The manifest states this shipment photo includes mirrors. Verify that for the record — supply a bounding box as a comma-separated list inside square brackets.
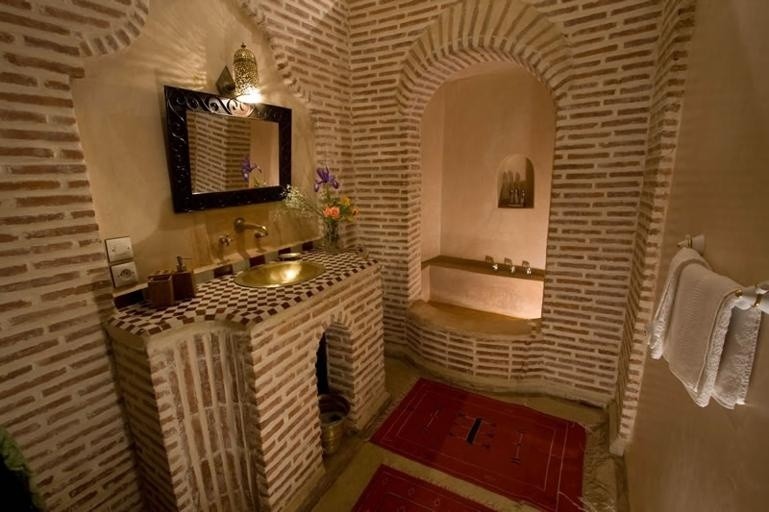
[164, 84, 292, 213]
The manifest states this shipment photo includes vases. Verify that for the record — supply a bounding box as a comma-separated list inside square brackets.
[315, 219, 348, 254]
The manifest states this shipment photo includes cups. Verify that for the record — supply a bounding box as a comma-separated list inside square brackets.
[148, 274, 175, 309]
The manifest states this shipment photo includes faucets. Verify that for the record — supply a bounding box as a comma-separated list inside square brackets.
[522, 261, 532, 275]
[485, 256, 498, 270]
[504, 258, 517, 274]
[233, 217, 268, 236]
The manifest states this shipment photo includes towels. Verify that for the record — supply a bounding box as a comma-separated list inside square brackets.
[644, 249, 762, 410]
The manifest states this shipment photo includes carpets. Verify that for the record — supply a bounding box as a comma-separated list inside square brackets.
[350, 464, 497, 512]
[366, 377, 586, 511]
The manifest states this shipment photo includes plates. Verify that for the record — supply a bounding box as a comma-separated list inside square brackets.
[278, 252, 300, 260]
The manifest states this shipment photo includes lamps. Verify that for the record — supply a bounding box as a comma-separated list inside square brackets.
[214, 41, 264, 105]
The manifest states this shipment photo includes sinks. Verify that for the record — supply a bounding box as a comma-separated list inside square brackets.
[233, 260, 326, 288]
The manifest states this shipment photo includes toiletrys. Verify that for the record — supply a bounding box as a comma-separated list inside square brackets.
[171, 255, 197, 299]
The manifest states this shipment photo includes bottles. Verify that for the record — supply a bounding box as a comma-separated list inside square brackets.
[509, 186, 526, 204]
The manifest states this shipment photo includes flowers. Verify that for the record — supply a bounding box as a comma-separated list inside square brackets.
[240, 158, 270, 188]
[281, 164, 357, 239]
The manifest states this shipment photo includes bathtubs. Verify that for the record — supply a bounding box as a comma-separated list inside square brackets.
[419, 265, 544, 320]
